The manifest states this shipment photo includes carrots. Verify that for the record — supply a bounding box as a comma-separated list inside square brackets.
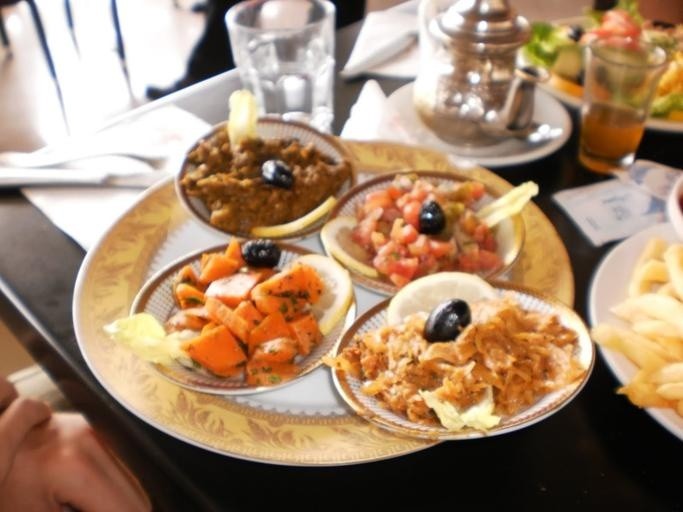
[167, 240, 324, 385]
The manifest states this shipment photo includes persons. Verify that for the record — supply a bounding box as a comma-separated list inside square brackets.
[1, 380, 152, 509]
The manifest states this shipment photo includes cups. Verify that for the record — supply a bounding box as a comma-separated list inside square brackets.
[224, 0, 338, 135]
[574, 33, 671, 176]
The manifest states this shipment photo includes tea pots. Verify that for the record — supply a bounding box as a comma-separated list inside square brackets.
[410, 0, 551, 157]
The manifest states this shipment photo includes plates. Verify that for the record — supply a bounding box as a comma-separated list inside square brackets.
[386, 79, 575, 169]
[587, 220, 683, 444]
[128, 235, 359, 397]
[321, 167, 528, 297]
[329, 278, 598, 442]
[173, 115, 360, 246]
[70, 138, 578, 473]
[513, 14, 682, 134]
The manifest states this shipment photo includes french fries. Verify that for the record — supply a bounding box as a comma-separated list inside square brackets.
[601, 239, 683, 419]
